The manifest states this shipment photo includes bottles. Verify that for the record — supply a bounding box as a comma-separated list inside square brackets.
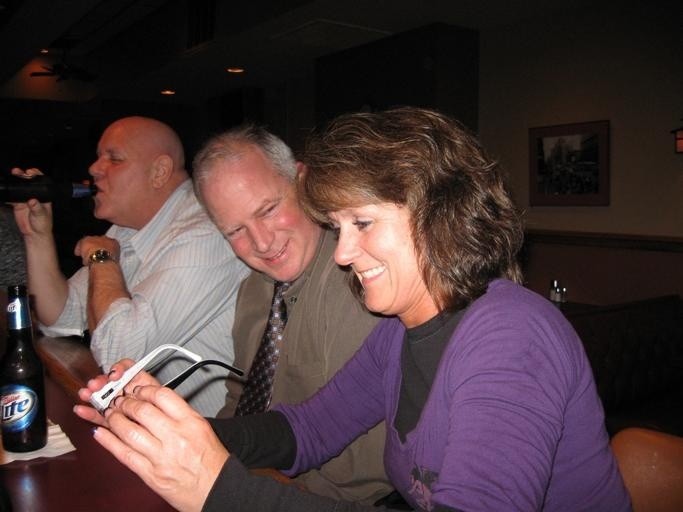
[548, 279, 567, 303]
[0, 175, 97, 205]
[0, 283, 47, 452]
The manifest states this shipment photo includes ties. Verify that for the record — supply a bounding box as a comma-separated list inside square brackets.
[234, 286, 288, 415]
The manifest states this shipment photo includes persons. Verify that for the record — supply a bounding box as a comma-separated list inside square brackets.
[192, 125, 394, 500]
[10, 117, 250, 419]
[72, 109, 630, 512]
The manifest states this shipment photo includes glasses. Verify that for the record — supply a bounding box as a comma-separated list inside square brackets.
[91, 343, 243, 416]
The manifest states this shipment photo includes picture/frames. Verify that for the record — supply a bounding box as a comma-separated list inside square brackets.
[524, 116, 615, 208]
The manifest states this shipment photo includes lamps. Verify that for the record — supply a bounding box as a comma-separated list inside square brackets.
[668, 126, 682, 154]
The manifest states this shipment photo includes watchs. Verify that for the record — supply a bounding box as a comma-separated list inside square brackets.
[88, 247, 117, 269]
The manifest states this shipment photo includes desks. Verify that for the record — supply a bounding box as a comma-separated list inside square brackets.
[0, 319, 334, 512]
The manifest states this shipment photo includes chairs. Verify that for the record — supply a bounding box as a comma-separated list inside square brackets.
[605, 426, 681, 510]
